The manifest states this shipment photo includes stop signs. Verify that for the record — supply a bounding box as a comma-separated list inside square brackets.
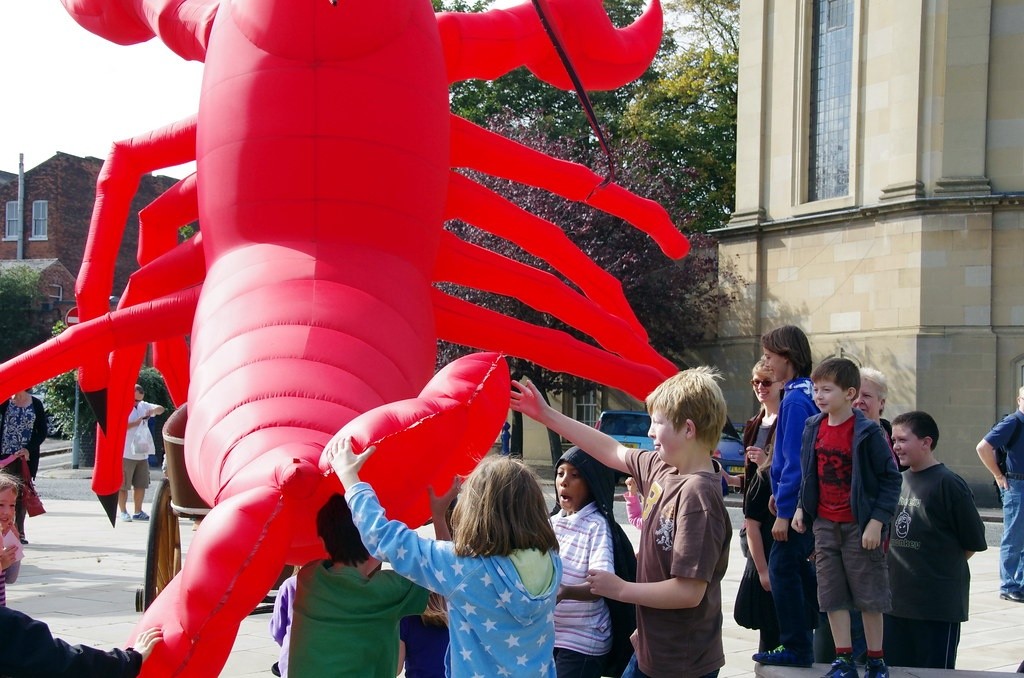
[65, 308, 79, 327]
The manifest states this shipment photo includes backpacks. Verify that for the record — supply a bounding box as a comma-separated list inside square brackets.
[993, 413, 1021, 502]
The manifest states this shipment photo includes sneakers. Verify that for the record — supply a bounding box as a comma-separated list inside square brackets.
[120, 512, 132, 522]
[821, 654, 859, 678]
[862, 656, 889, 678]
[752, 645, 812, 667]
[132, 512, 149, 521]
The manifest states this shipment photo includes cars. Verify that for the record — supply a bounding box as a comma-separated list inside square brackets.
[32, 388, 66, 437]
[710, 422, 746, 489]
[595, 410, 654, 483]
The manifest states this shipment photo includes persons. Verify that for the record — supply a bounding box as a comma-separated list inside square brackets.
[0, 387, 47, 544]
[396, 592, 448, 678]
[0, 606, 163, 678]
[288, 493, 429, 678]
[624, 476, 642, 529]
[510, 364, 728, 678]
[326, 434, 563, 678]
[734, 324, 988, 678]
[548, 445, 637, 678]
[270, 565, 302, 678]
[118, 384, 165, 522]
[975, 386, 1024, 603]
[0, 464, 31, 606]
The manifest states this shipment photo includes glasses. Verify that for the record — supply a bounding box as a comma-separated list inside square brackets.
[750, 379, 781, 388]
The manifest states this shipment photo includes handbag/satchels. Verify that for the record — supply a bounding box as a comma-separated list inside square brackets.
[19, 457, 46, 517]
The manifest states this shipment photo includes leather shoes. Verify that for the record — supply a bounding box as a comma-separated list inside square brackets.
[1000, 592, 1024, 602]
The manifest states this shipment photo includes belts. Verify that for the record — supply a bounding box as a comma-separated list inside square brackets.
[1007, 474, 1024, 480]
